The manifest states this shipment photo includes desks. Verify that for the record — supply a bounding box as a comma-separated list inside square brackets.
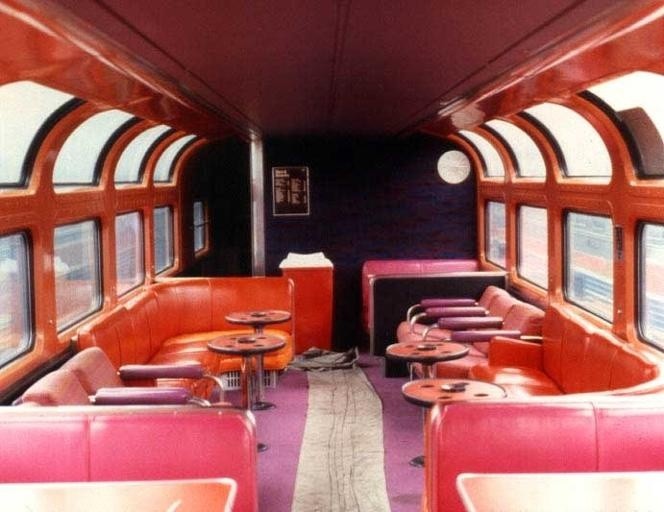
[401, 376, 508, 466]
[385, 339, 470, 468]
[205, 309, 290, 453]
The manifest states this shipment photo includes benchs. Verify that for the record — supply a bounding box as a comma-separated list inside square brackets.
[362, 259, 479, 335]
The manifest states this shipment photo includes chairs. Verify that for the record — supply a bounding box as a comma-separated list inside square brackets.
[12, 346, 233, 409]
[395, 284, 544, 382]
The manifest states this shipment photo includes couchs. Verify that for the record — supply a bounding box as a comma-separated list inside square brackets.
[422, 301, 664, 511]
[74, 278, 295, 400]
